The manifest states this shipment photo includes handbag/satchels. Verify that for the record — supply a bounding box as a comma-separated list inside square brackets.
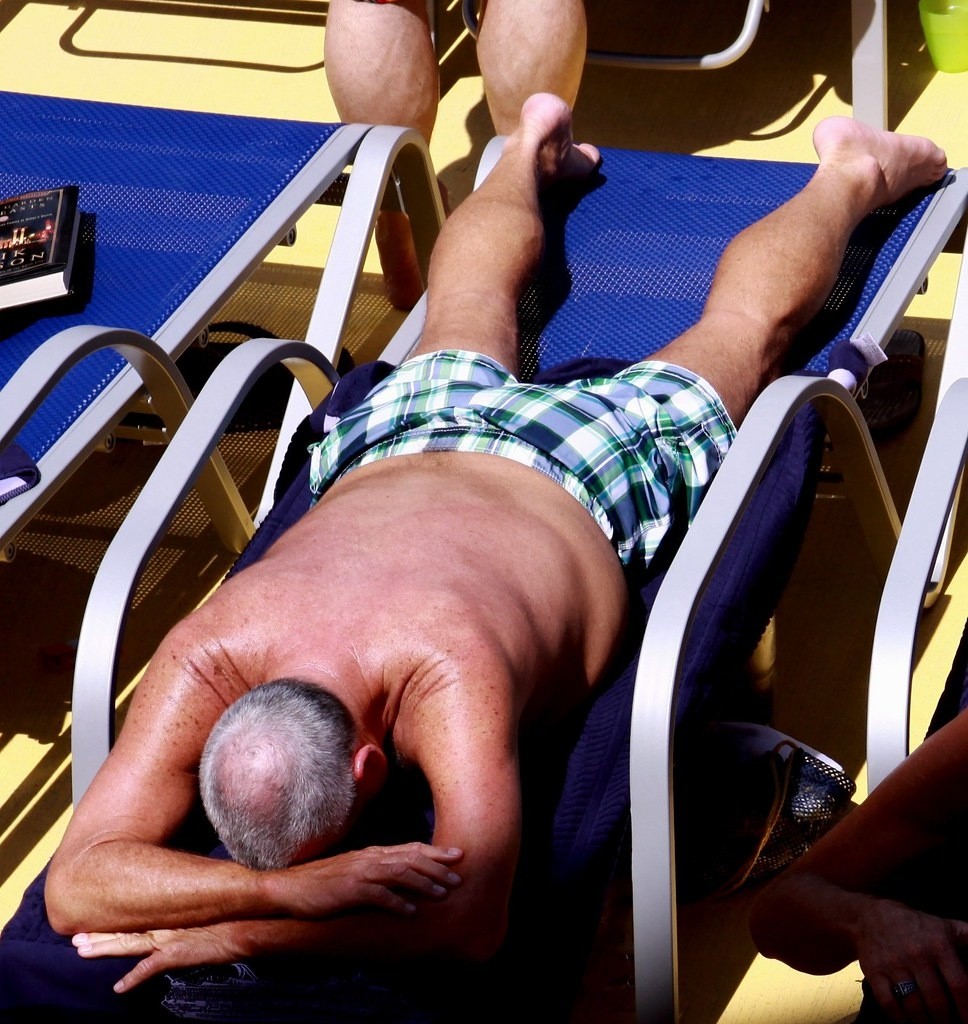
[673, 719, 844, 896]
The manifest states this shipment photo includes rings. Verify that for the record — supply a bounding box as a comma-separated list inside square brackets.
[892, 981, 917, 998]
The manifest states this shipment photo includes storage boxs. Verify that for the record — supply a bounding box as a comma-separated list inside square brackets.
[1, 185, 84, 310]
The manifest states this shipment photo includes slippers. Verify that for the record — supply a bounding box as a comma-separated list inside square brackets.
[855, 329, 925, 443]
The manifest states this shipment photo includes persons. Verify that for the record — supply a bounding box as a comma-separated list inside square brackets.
[745, 709, 968, 1024]
[324, 1, 587, 317]
[44, 90, 943, 996]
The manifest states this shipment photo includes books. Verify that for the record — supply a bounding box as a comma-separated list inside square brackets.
[0, 186, 82, 310]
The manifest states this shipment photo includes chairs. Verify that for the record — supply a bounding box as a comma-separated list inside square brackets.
[0, 89, 968, 1024]
